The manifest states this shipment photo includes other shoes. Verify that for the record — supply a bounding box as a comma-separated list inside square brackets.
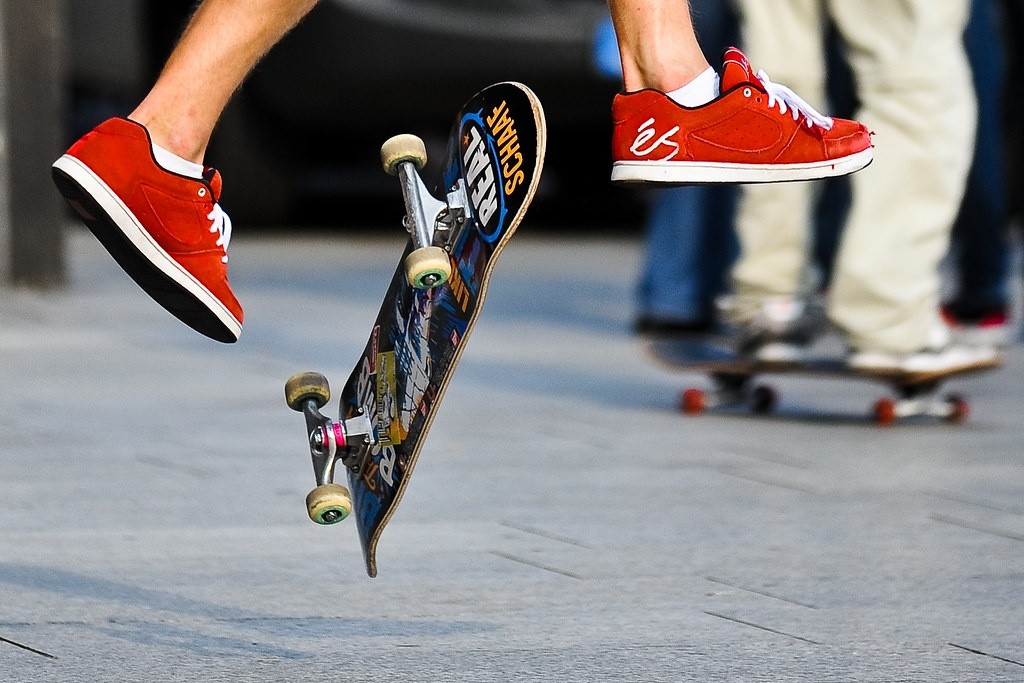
[741, 311, 844, 361]
[938, 302, 1015, 350]
[850, 333, 995, 368]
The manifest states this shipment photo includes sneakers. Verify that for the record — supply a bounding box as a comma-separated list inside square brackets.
[50, 114, 246, 345]
[609, 46, 874, 187]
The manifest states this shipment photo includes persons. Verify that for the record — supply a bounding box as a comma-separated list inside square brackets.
[51, 0, 879, 345]
[596, 0, 1024, 374]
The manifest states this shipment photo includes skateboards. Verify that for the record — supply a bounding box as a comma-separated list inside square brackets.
[283, 81, 549, 578]
[642, 338, 1001, 425]
